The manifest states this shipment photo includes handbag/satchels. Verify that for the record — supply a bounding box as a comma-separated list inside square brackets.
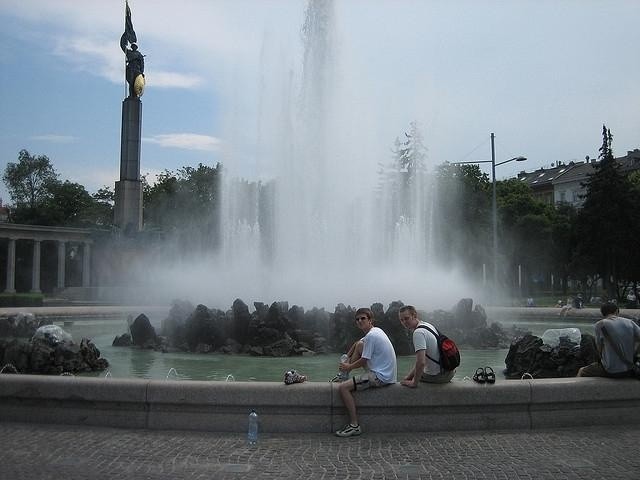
[629, 358, 640, 378]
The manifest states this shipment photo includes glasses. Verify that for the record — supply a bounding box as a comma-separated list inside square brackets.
[356, 316, 366, 322]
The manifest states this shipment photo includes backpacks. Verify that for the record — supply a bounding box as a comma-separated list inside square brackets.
[415, 321, 460, 371]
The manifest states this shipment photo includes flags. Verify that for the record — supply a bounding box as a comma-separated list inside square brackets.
[125, 4, 137, 43]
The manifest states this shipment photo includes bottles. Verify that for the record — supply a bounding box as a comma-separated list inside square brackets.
[248, 409, 258, 440]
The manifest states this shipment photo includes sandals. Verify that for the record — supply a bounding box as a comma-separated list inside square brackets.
[472, 367, 486, 383]
[484, 366, 496, 383]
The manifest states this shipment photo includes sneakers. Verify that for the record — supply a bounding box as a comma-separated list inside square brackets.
[335, 423, 362, 437]
[328, 373, 348, 383]
[283, 369, 307, 385]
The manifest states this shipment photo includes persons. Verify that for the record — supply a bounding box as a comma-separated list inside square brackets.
[521, 288, 640, 309]
[326, 307, 398, 438]
[398, 305, 457, 388]
[120, 34, 144, 98]
[577, 301, 640, 378]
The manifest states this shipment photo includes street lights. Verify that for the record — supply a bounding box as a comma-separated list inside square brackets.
[451, 133, 531, 308]
